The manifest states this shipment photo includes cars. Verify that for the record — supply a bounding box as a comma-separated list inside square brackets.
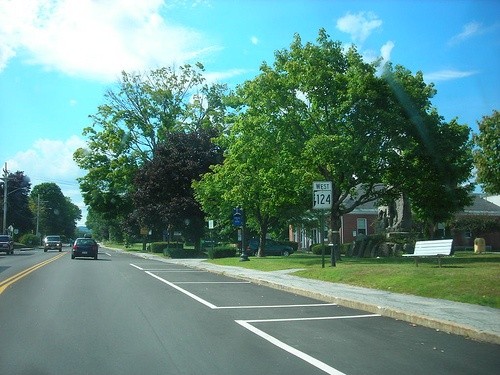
[71, 238, 97, 260]
[240, 238, 294, 256]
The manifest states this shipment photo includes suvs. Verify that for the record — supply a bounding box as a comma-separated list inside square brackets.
[44, 236, 62, 252]
[0, 235, 14, 254]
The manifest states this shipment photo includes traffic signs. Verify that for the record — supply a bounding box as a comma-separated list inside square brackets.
[313, 181, 332, 209]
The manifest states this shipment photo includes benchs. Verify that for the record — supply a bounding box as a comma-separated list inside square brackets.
[402, 239, 454, 268]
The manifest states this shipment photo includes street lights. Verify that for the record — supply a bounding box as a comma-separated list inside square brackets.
[3, 187, 28, 234]
[36, 208, 52, 235]
